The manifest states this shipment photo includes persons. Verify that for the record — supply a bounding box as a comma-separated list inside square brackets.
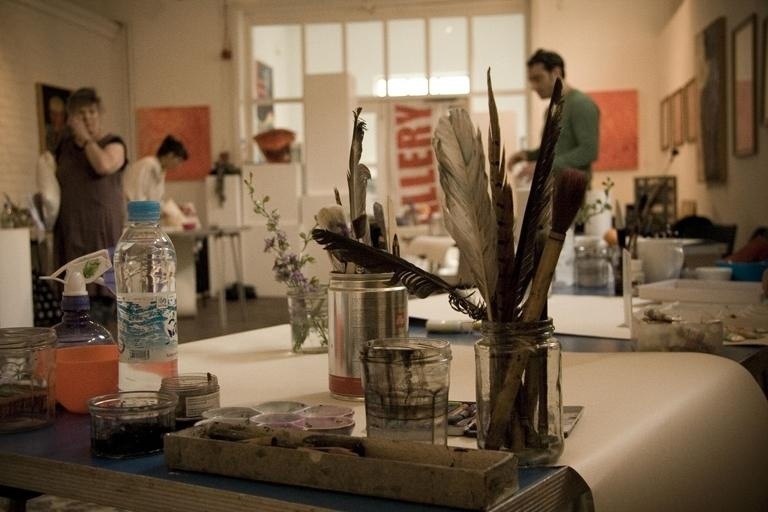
[508, 48, 599, 233]
[45, 87, 128, 302]
[124, 133, 189, 213]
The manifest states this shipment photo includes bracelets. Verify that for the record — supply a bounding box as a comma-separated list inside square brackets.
[80, 136, 92, 148]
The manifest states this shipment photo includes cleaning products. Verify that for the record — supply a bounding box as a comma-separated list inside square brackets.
[38, 248, 112, 349]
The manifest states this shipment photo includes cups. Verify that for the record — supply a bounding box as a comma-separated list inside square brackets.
[0, 325, 59, 434]
[572, 246, 617, 298]
[358, 336, 455, 448]
[87, 390, 179, 461]
[159, 374, 221, 430]
[695, 267, 733, 281]
[194, 399, 356, 438]
[639, 239, 684, 284]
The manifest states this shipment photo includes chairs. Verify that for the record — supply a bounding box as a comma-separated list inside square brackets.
[669, 214, 738, 258]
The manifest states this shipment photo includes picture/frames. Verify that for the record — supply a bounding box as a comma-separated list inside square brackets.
[659, 13, 768, 182]
[34, 81, 75, 155]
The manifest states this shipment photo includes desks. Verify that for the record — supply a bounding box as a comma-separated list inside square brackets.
[0, 225, 52, 328]
[166, 224, 253, 328]
[0, 281, 767, 512]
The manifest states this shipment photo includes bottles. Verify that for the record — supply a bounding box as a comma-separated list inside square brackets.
[114, 199, 179, 392]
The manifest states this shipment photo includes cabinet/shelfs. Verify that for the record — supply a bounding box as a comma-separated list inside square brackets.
[242, 72, 388, 298]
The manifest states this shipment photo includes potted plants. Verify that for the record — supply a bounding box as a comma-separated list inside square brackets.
[239, 172, 332, 354]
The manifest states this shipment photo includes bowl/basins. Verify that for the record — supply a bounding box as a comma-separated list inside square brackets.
[629, 320, 724, 356]
[34, 343, 121, 416]
